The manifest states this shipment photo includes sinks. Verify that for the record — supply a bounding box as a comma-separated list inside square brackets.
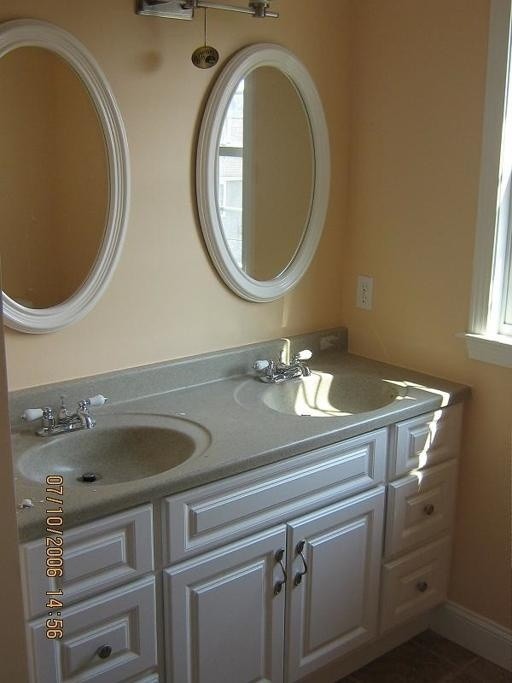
[233, 370, 409, 419]
[14, 410, 213, 486]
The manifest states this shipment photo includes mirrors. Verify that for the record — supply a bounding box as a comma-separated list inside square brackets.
[189, 37, 333, 310]
[2, 17, 134, 336]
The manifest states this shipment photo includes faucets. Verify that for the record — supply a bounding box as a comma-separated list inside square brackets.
[56, 393, 95, 430]
[275, 349, 313, 378]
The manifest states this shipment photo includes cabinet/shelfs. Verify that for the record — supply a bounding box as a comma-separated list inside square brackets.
[381, 400, 461, 632]
[163, 424, 391, 683]
[20, 491, 166, 681]
